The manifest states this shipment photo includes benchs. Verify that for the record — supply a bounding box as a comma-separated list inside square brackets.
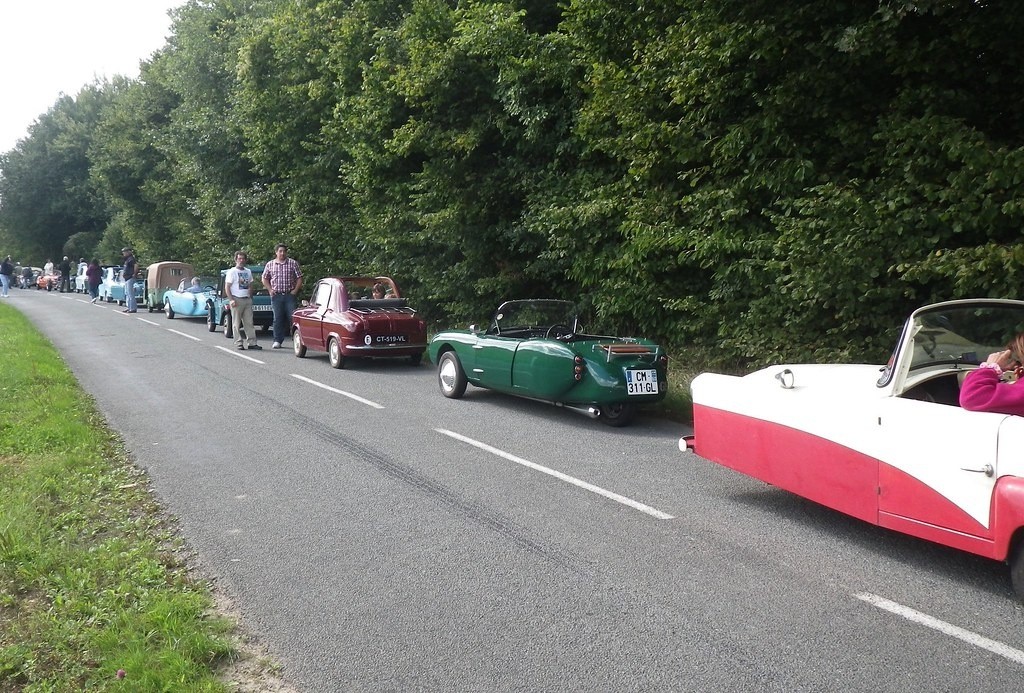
[349, 297, 408, 308]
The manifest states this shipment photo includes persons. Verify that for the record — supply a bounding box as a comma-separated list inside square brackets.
[0, 257, 14, 297]
[121, 247, 139, 313]
[12, 261, 22, 280]
[262, 244, 304, 349]
[43, 258, 54, 275]
[86, 257, 104, 304]
[59, 256, 73, 293]
[372, 283, 387, 299]
[187, 277, 205, 293]
[959, 333, 1024, 417]
[79, 258, 86, 275]
[20, 264, 33, 289]
[225, 252, 262, 350]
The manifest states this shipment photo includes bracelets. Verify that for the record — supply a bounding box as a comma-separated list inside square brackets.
[980, 362, 1003, 378]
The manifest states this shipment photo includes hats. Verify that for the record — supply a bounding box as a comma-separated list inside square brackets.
[122, 247, 131, 252]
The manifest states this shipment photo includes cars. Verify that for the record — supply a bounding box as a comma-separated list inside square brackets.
[111, 270, 145, 306]
[429, 299, 670, 427]
[163, 278, 218, 319]
[678, 297, 1024, 606]
[289, 276, 427, 369]
[11, 262, 124, 303]
[143, 261, 194, 313]
[204, 266, 274, 338]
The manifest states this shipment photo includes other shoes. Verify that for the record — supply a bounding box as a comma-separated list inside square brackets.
[272, 342, 281, 349]
[248, 345, 262, 350]
[121, 308, 137, 313]
[237, 345, 244, 350]
